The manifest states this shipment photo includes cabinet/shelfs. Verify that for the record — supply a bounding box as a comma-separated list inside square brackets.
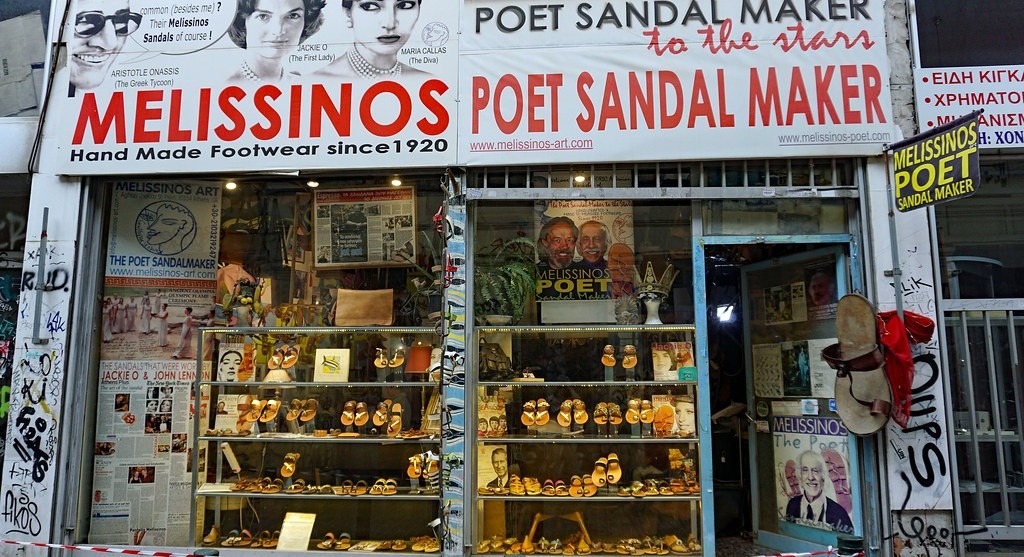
[190, 325, 703, 557]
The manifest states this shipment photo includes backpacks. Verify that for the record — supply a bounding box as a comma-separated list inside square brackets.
[478, 337, 515, 381]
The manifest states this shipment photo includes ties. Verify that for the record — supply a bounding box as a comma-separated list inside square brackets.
[498, 479, 503, 487]
[806, 504, 813, 520]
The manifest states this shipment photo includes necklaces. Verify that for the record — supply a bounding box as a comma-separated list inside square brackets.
[240, 58, 287, 85]
[346, 42, 402, 82]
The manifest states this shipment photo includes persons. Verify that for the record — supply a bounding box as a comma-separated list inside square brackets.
[102, 289, 242, 483]
[531, 176, 610, 302]
[224, 0, 328, 86]
[307, 0, 438, 88]
[343, 203, 367, 232]
[652, 342, 698, 439]
[760, 269, 853, 536]
[69, 0, 141, 90]
[477, 415, 510, 488]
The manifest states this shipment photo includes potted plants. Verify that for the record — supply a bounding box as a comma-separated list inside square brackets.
[395, 229, 548, 325]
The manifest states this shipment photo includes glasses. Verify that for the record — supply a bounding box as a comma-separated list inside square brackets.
[73, 12, 142, 38]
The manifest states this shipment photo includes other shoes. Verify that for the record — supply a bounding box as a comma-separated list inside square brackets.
[653, 406, 674, 438]
[785, 460, 801, 496]
[821, 447, 852, 513]
[607, 243, 635, 298]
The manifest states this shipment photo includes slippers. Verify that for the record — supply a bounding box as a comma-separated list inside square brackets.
[375, 535, 441, 552]
[389, 349, 406, 367]
[432, 167, 468, 551]
[202, 524, 281, 547]
[281, 344, 300, 368]
[626, 398, 641, 424]
[601, 344, 616, 367]
[535, 398, 550, 426]
[593, 403, 608, 425]
[373, 347, 388, 368]
[335, 532, 350, 550]
[607, 402, 622, 425]
[476, 532, 701, 556]
[316, 532, 337, 550]
[573, 399, 588, 425]
[521, 400, 536, 426]
[478, 453, 702, 498]
[639, 400, 655, 424]
[822, 293, 892, 437]
[622, 344, 637, 368]
[268, 344, 289, 370]
[203, 394, 434, 440]
[557, 400, 572, 427]
[229, 450, 440, 494]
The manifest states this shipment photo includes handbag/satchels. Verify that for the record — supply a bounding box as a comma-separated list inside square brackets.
[323, 288, 395, 327]
